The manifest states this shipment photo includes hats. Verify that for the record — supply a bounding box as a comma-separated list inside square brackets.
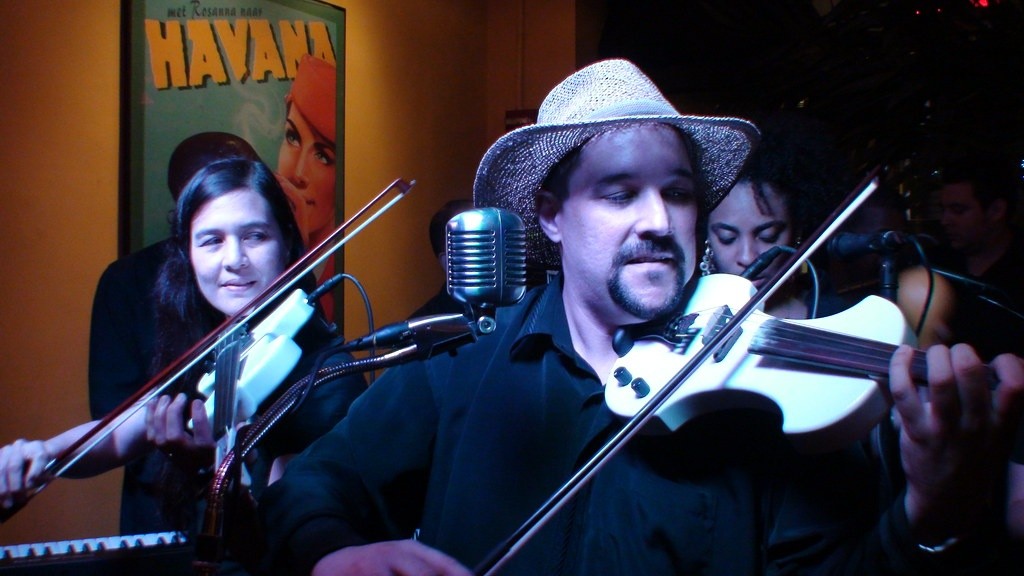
[472, 57, 763, 272]
[285, 52, 336, 145]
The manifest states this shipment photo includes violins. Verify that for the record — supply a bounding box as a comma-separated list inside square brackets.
[605, 273, 1001, 455]
[185, 286, 315, 493]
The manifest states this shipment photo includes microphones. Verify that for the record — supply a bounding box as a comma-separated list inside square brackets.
[446, 207, 527, 332]
[825, 229, 905, 262]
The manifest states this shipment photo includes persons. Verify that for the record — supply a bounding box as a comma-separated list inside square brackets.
[0, 132, 368, 536]
[256, 57, 1024, 576]
[407, 197, 476, 318]
[278, 55, 336, 322]
[698, 114, 1024, 538]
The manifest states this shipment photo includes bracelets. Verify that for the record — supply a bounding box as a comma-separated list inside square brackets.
[918, 538, 958, 553]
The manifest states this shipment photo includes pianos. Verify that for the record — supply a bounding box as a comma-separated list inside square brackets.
[0, 531, 186, 576]
